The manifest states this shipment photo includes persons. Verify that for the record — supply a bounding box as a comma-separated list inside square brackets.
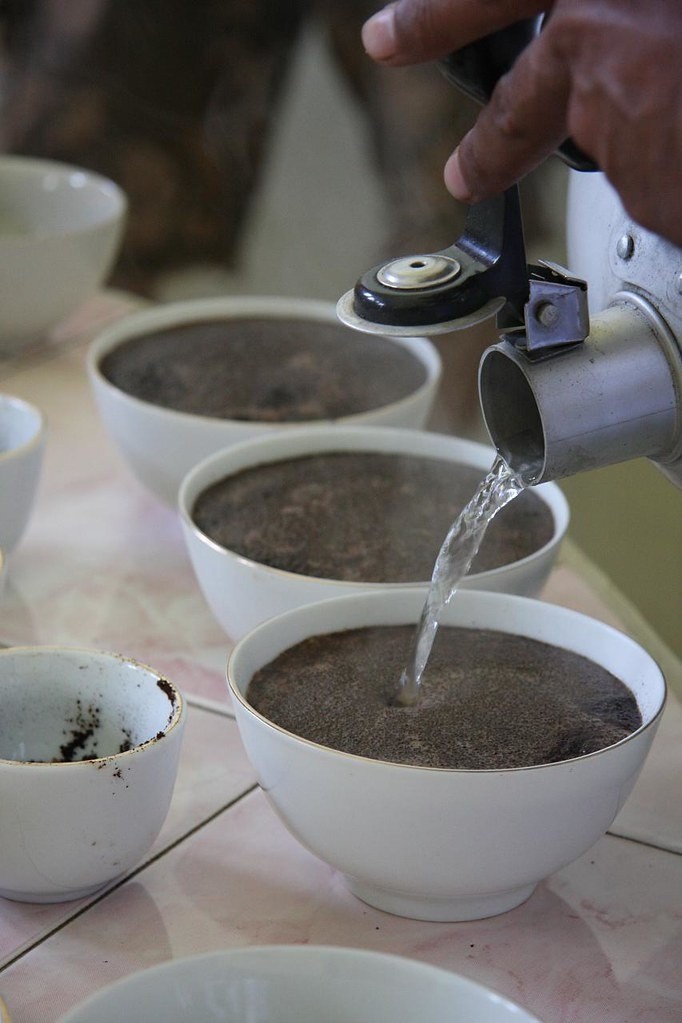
[0, 0, 682, 438]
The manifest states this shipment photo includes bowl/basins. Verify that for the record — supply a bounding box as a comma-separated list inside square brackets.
[87, 292, 443, 516]
[0, 644, 181, 904]
[174, 428, 571, 648]
[224, 588, 669, 924]
[0, 392, 46, 567]
[0, 157, 129, 358]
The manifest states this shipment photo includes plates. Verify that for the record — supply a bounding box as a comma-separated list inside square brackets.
[51, 944, 544, 1023]
